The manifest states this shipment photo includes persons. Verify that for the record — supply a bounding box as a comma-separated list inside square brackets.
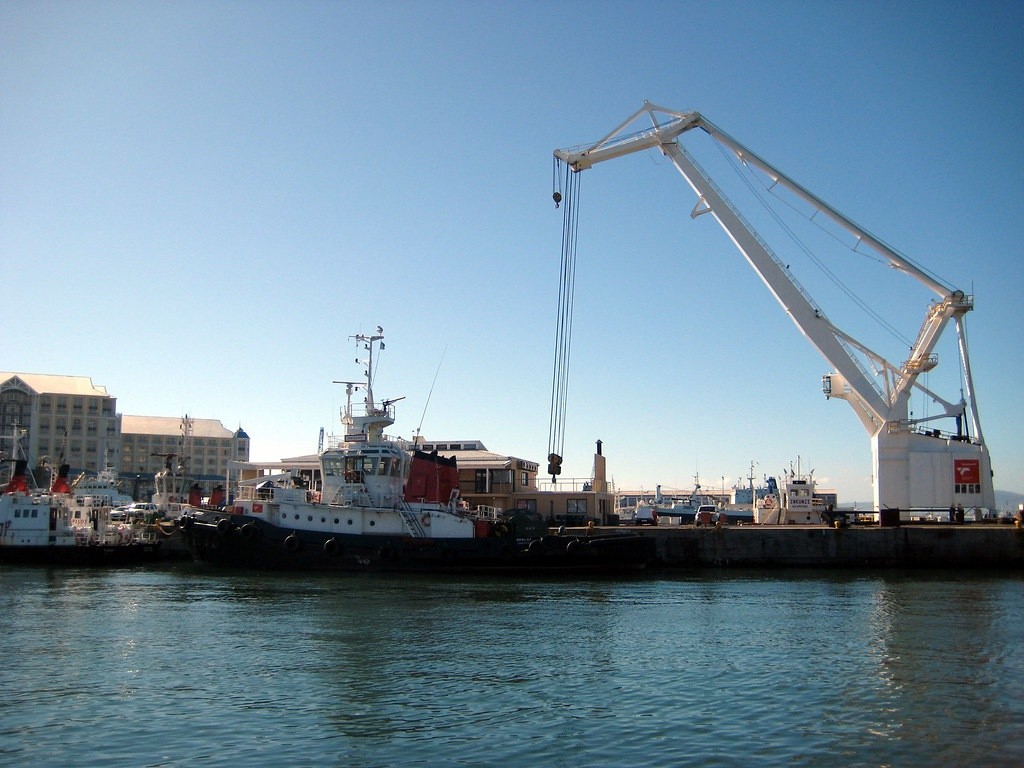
[634, 513, 638, 526]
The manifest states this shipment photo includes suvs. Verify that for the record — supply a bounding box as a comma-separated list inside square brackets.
[694, 505, 721, 527]
[125, 501, 159, 520]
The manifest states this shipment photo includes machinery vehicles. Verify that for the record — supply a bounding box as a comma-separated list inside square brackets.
[544, 99, 998, 526]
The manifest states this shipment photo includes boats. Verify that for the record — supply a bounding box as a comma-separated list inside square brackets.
[612, 453, 837, 526]
[0, 418, 188, 567]
[173, 324, 510, 576]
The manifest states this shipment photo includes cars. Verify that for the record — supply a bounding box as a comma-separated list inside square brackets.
[110, 506, 129, 521]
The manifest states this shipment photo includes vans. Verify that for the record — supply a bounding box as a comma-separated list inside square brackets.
[164, 503, 191, 520]
